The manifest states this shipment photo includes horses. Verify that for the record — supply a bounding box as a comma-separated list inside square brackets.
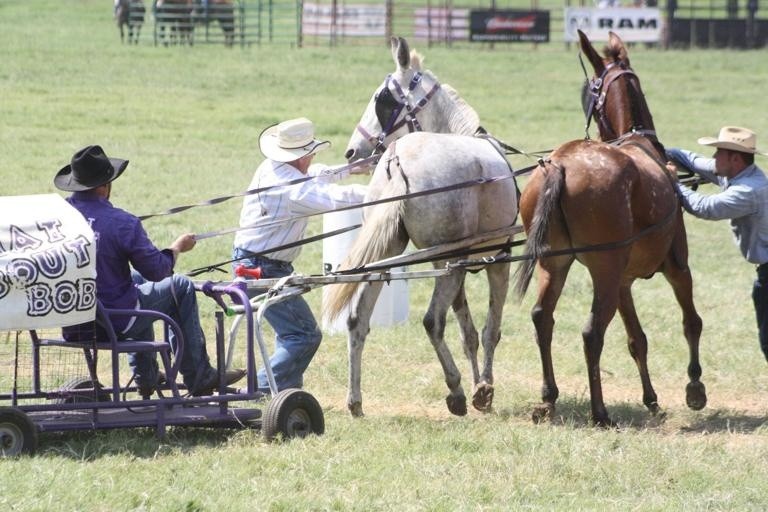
[153, 1, 234, 49]
[116, 0, 146, 46]
[502, 29, 708, 429]
[313, 36, 522, 419]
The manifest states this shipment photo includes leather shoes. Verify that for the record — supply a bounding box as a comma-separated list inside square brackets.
[137, 370, 167, 395]
[188, 366, 249, 397]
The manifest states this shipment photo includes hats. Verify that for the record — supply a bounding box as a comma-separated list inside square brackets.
[257, 116, 332, 163]
[52, 144, 130, 192]
[696, 125, 768, 157]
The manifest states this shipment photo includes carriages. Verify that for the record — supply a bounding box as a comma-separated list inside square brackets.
[0, 28, 707, 459]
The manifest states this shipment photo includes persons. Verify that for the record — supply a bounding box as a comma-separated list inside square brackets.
[53, 144, 247, 398]
[231, 117, 369, 396]
[662, 126, 768, 366]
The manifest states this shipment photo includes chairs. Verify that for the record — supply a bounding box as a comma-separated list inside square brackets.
[29, 298, 185, 403]
